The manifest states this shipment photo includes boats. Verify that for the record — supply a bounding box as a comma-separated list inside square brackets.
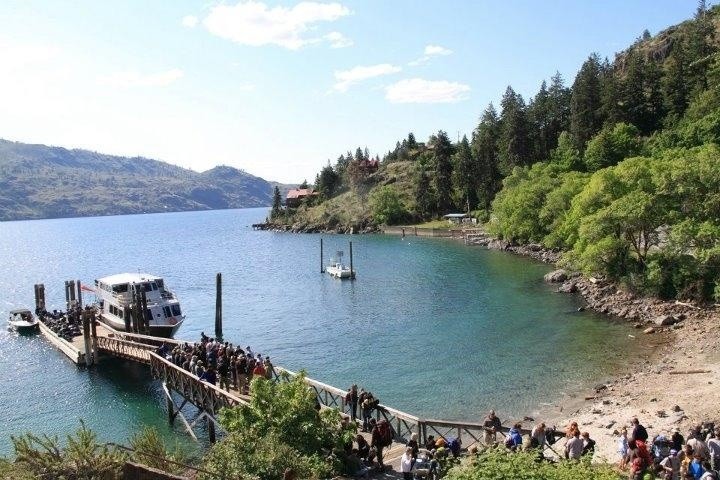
[326, 248, 356, 279]
[7, 309, 39, 332]
[92, 271, 186, 339]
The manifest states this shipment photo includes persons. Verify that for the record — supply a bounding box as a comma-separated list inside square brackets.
[157, 332, 273, 394]
[25, 298, 92, 341]
[620, 418, 720, 480]
[346, 385, 595, 480]
